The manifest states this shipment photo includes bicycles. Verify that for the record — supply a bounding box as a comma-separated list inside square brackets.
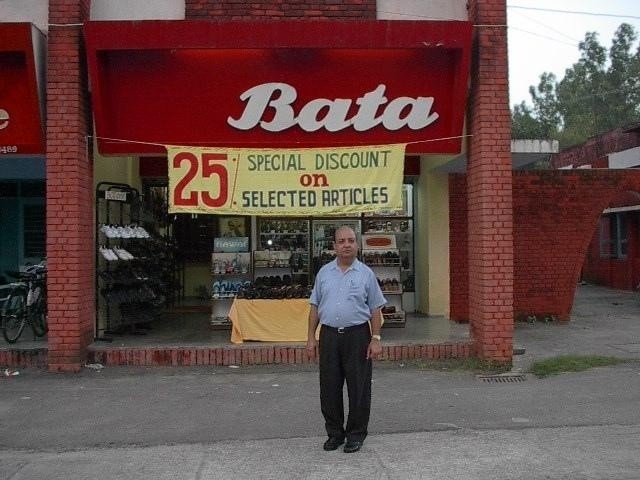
[0, 253, 49, 345]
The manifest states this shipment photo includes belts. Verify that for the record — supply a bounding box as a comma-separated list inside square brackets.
[323, 322, 368, 335]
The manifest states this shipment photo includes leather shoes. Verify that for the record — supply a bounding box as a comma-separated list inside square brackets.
[343, 440, 363, 453]
[323, 435, 345, 451]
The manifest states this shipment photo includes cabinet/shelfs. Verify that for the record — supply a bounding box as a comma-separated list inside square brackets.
[211, 185, 416, 329]
[94, 181, 168, 342]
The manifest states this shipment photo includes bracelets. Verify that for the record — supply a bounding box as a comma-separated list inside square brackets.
[371, 334, 382, 341]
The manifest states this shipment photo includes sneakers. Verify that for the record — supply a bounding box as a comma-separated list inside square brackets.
[99, 223, 151, 239]
[213, 258, 248, 275]
[212, 274, 312, 299]
[365, 251, 409, 270]
[377, 277, 414, 292]
[99, 244, 146, 262]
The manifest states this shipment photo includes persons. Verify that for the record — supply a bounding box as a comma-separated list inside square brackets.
[221, 220, 244, 238]
[305, 224, 389, 454]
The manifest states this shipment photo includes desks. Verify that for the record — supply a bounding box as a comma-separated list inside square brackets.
[229, 295, 323, 344]
